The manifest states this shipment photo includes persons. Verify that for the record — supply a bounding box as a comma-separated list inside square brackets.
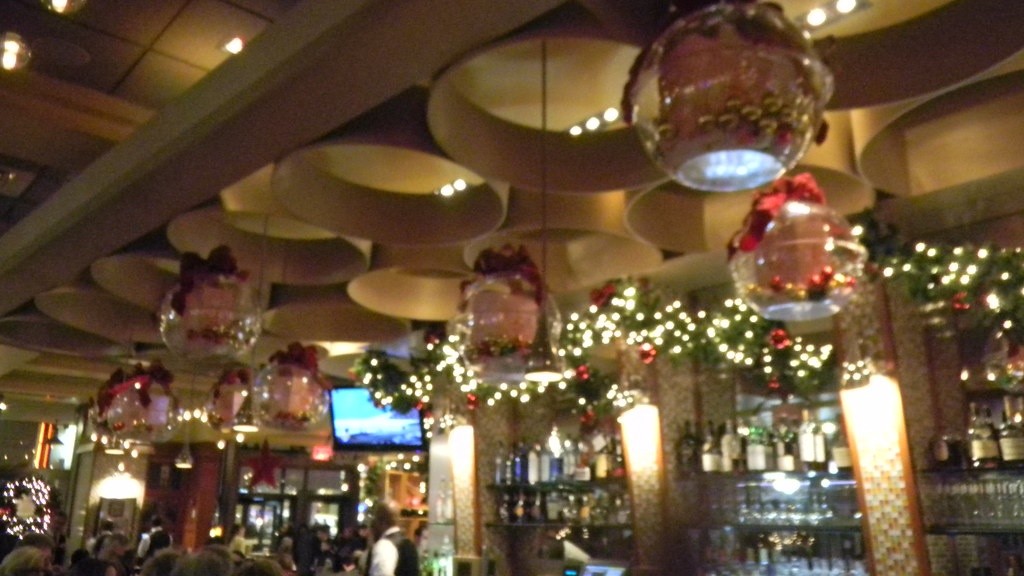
[366, 503, 422, 576]
[0, 475, 429, 575]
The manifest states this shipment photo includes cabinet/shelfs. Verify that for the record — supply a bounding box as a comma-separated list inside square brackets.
[672, 469, 862, 535]
[483, 475, 630, 532]
[383, 469, 427, 540]
[919, 461, 1024, 532]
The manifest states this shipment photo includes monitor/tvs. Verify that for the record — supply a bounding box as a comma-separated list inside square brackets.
[326, 383, 430, 452]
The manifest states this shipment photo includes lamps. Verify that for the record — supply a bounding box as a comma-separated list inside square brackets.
[524, 40, 565, 384]
[107, 325, 134, 455]
[232, 163, 271, 431]
[174, 386, 193, 469]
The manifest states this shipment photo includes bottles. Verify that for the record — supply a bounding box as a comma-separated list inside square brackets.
[926, 401, 1024, 473]
[489, 436, 631, 526]
[676, 418, 832, 475]
